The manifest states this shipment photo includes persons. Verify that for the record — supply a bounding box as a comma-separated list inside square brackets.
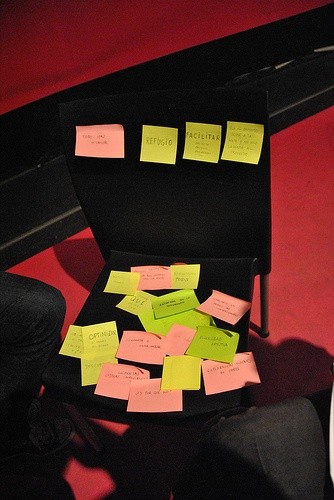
[0, 266, 68, 454]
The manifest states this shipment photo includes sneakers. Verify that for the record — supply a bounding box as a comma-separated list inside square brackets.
[0, 416, 74, 462]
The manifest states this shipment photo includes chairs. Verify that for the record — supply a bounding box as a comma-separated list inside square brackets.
[39, 85, 272, 421]
